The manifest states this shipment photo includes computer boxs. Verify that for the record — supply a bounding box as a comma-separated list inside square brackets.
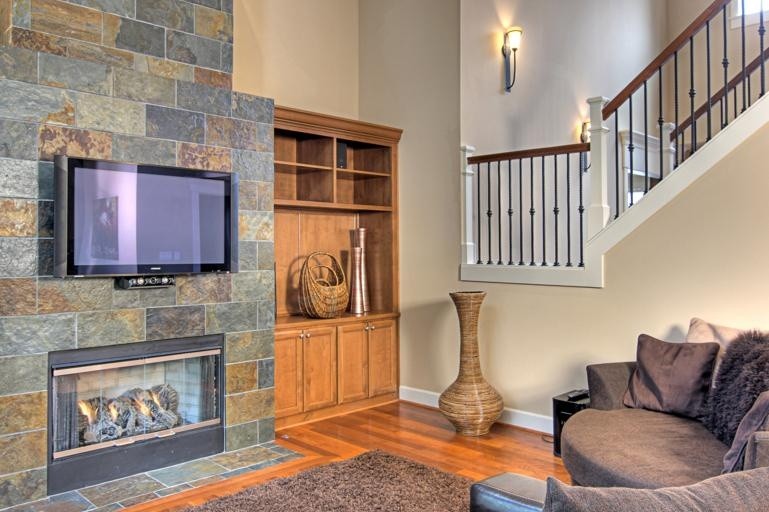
[552, 389, 590, 458]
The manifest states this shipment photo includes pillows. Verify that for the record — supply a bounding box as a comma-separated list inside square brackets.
[620, 315, 744, 424]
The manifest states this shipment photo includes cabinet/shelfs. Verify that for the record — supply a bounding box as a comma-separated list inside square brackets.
[273, 105, 403, 433]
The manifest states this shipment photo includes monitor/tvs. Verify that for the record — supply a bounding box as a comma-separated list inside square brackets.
[53, 154, 238, 288]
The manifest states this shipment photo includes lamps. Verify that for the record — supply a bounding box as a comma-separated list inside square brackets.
[503, 26, 523, 91]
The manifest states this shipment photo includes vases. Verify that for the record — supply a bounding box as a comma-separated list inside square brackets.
[438, 290, 505, 439]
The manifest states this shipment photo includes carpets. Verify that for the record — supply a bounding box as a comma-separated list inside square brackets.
[172, 446, 476, 512]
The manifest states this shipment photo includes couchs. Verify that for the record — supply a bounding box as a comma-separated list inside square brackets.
[545, 332, 768, 512]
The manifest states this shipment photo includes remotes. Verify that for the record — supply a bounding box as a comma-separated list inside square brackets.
[567, 390, 588, 400]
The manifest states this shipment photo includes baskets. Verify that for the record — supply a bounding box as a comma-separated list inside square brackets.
[297, 252, 349, 319]
